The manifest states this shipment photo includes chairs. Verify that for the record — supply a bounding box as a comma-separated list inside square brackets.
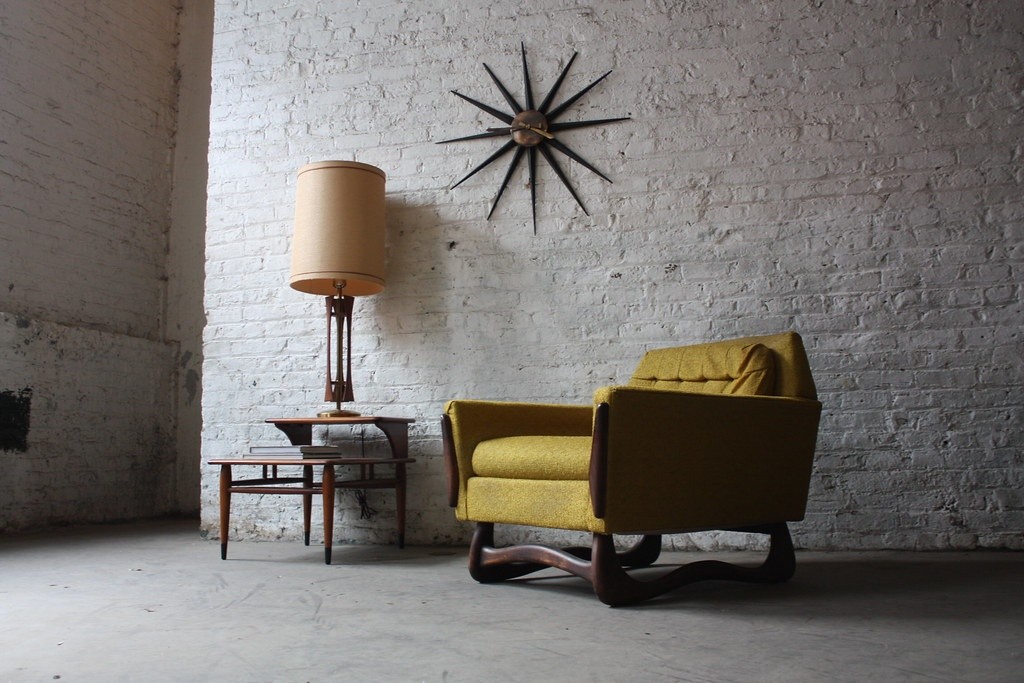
[440, 330, 823, 608]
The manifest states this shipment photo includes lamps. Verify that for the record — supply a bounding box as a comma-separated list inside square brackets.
[288, 160, 389, 418]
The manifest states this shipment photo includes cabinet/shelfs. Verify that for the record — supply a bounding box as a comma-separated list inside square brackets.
[207, 417, 416, 565]
